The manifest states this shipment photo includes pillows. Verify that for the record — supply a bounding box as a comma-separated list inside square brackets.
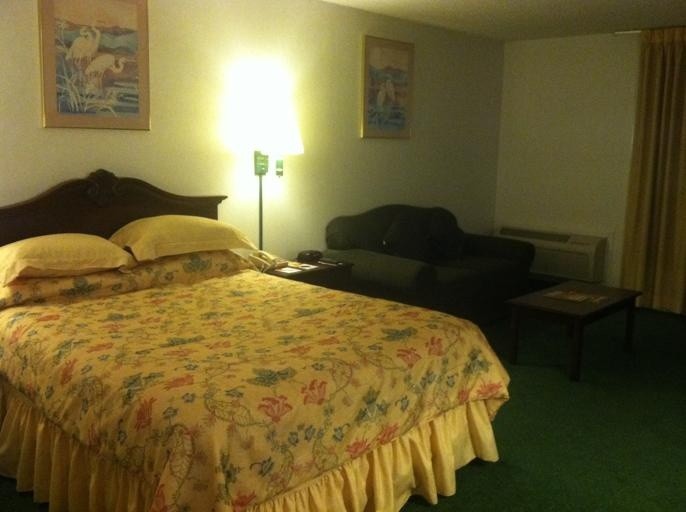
[5, 269, 133, 306]
[132, 250, 245, 294]
[108, 214, 252, 264]
[4, 232, 131, 277]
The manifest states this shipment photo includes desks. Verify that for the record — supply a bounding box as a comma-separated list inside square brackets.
[508, 282, 644, 386]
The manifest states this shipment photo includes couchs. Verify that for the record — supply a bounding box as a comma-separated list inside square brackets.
[327, 205, 534, 331]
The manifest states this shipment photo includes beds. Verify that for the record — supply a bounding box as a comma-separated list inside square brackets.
[0, 166, 512, 512]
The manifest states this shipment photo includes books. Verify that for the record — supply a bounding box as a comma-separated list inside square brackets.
[543, 290, 607, 304]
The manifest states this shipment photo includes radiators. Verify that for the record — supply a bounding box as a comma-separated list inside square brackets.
[495, 225, 607, 282]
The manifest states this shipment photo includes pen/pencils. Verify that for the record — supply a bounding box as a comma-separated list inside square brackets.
[300, 265, 310, 267]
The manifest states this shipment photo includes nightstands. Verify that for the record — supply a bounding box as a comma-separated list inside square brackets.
[269, 257, 354, 295]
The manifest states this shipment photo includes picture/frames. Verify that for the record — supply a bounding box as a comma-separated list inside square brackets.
[36, 0, 150, 131]
[360, 33, 415, 142]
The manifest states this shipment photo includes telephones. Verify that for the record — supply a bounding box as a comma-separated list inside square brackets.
[249, 251, 289, 270]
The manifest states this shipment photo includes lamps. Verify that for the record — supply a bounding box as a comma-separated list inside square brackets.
[219, 64, 305, 250]
